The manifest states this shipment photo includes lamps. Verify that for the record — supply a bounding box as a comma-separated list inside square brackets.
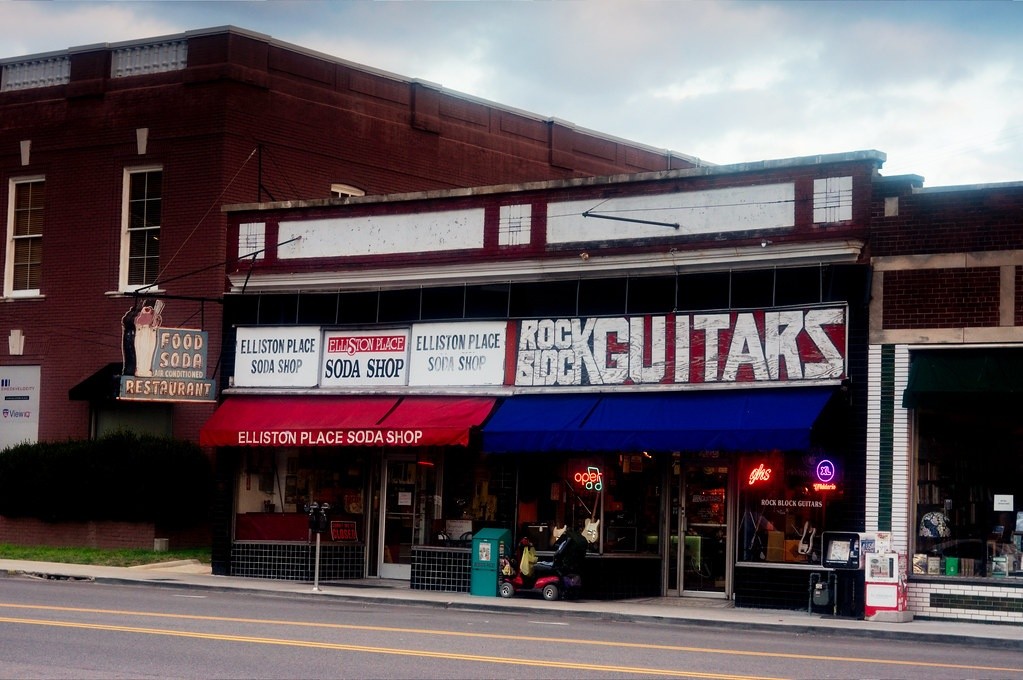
[760, 239, 773, 247]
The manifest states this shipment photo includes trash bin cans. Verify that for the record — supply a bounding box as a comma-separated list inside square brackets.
[470, 528, 510, 597]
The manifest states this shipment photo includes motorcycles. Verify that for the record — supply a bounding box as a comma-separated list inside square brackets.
[498, 531, 591, 601]
